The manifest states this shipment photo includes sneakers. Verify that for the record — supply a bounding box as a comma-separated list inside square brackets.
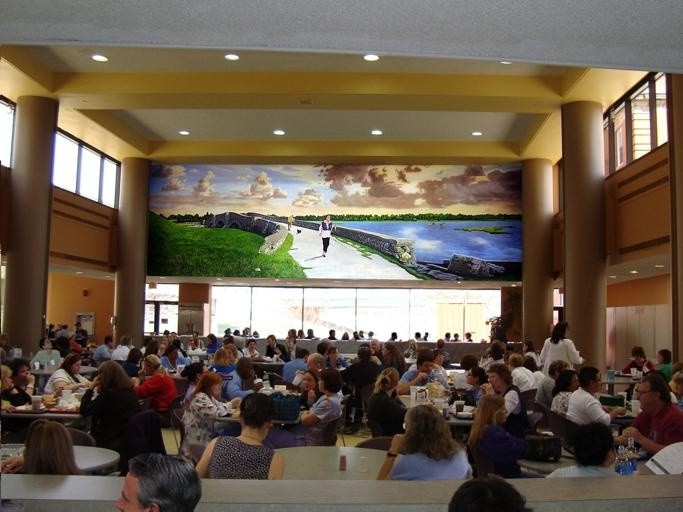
[337, 421, 360, 435]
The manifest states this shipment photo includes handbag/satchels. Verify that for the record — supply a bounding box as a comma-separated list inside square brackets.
[268, 392, 303, 422]
[597, 395, 626, 407]
[522, 434, 562, 462]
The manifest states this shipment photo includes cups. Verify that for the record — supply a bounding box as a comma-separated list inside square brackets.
[254, 378, 262, 384]
[31, 396, 42, 410]
[357, 456, 369, 473]
[33, 361, 40, 371]
[55, 389, 81, 412]
[630, 400, 641, 417]
[454, 400, 465, 416]
[630, 368, 637, 376]
[618, 391, 628, 408]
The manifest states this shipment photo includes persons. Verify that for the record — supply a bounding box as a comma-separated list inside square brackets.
[318, 215, 336, 257]
[287, 214, 293, 230]
[2, 320, 682, 512]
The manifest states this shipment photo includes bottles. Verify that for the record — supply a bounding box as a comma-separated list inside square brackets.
[339, 455, 346, 471]
[612, 436, 638, 475]
